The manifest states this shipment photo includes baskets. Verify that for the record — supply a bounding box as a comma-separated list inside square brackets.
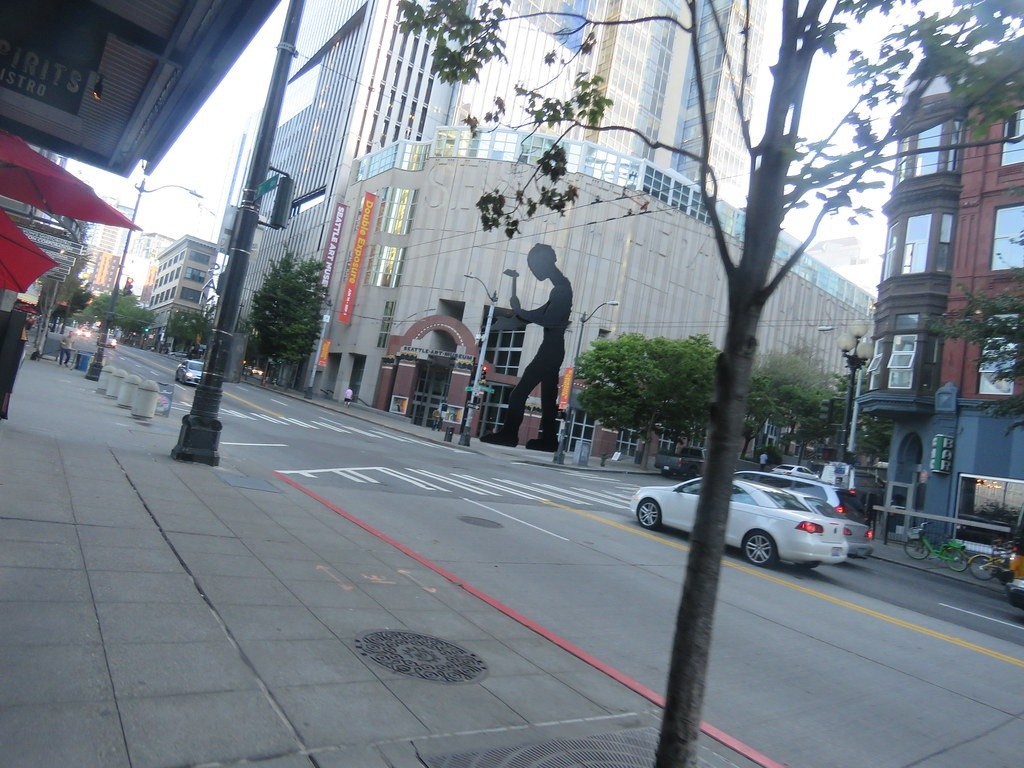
[907, 527, 920, 539]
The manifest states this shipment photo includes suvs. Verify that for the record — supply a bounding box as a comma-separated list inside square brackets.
[734, 470, 874, 560]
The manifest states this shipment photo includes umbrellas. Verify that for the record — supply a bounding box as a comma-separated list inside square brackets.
[0, 131, 143, 232]
[0, 207, 61, 294]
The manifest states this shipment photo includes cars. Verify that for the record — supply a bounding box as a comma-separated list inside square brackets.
[79, 328, 90, 337]
[630, 474, 848, 572]
[780, 486, 874, 560]
[97, 333, 117, 348]
[1005, 500, 1024, 611]
[175, 359, 204, 386]
[769, 464, 820, 481]
[167, 350, 188, 358]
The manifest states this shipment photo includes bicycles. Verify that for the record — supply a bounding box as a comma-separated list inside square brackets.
[904, 521, 970, 572]
[966, 539, 1014, 581]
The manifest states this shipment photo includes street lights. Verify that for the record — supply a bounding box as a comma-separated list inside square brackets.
[553, 300, 620, 464]
[458, 273, 500, 446]
[837, 318, 875, 463]
[85, 177, 204, 382]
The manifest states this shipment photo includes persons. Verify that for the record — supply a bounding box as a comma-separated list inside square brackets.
[432, 409, 442, 432]
[759, 452, 768, 471]
[59, 331, 75, 367]
[344, 387, 353, 407]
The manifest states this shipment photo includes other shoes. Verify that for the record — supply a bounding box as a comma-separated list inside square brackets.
[64, 362, 68, 367]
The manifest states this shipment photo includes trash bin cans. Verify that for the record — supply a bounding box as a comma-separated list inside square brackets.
[444, 426, 455, 441]
[76, 351, 92, 371]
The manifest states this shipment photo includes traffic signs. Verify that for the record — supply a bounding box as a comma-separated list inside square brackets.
[466, 403, 480, 410]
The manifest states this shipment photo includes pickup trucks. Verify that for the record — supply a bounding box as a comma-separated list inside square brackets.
[653, 445, 708, 480]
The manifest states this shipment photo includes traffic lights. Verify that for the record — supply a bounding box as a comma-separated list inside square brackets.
[122, 277, 134, 296]
[481, 366, 487, 380]
